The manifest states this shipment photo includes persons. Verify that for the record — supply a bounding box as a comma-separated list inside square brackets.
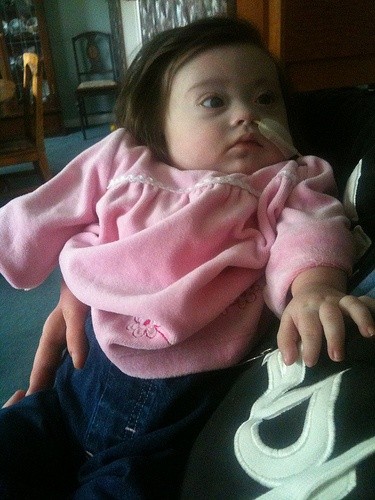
[0, 106, 374, 500]
[0, 9, 374, 500]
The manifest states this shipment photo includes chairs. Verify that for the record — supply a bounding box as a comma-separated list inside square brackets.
[71, 31, 125, 140]
[0, 53, 56, 202]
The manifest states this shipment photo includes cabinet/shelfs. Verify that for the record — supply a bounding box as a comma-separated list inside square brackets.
[1, 1, 61, 139]
